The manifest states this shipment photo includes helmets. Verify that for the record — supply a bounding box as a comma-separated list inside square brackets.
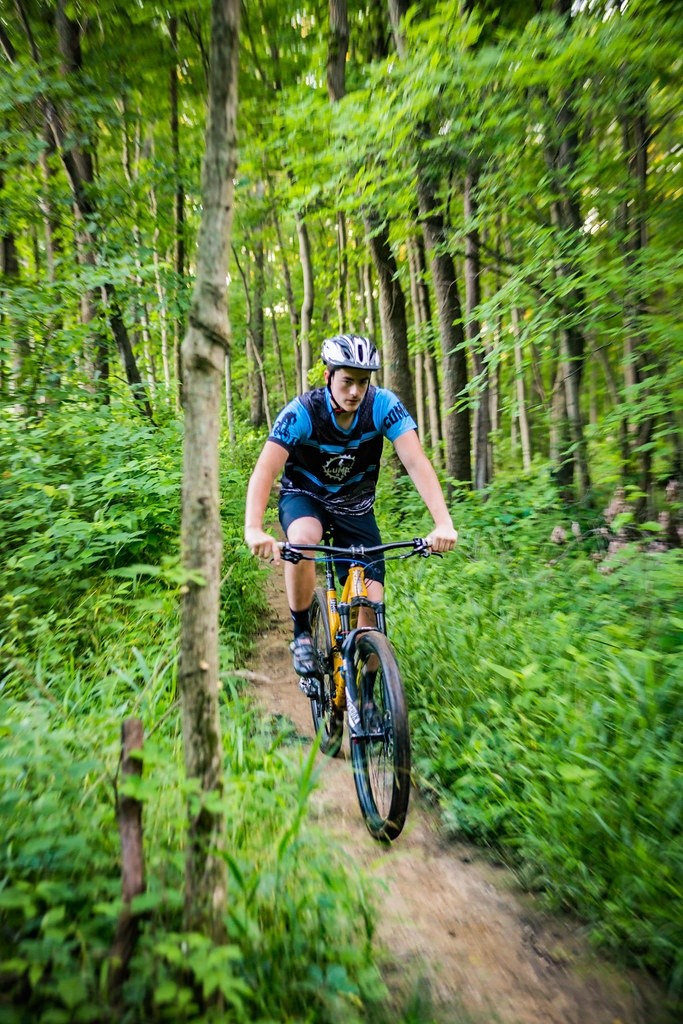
[320, 333, 381, 371]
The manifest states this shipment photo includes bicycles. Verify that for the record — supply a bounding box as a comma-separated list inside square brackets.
[266, 538, 447, 843]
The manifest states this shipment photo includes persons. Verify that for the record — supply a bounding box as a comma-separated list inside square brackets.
[244, 334, 458, 733]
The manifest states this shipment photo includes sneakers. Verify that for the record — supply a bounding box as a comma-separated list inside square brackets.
[362, 701, 382, 732]
[288, 632, 319, 678]
